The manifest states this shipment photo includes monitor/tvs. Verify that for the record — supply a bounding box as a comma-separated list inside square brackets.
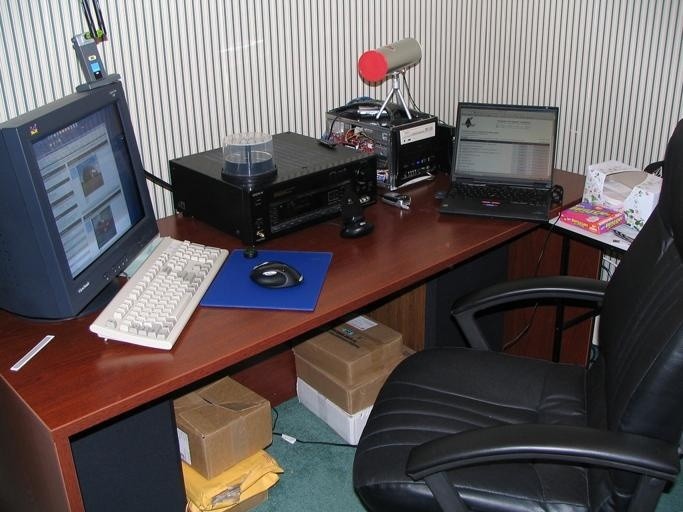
[0, 81, 159, 323]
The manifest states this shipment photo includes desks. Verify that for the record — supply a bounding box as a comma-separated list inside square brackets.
[542, 198, 650, 366]
[1, 166, 607, 512]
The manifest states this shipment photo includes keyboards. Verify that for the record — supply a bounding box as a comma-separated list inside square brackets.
[90, 236, 229, 350]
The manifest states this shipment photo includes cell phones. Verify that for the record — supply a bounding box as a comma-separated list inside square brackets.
[382, 191, 411, 210]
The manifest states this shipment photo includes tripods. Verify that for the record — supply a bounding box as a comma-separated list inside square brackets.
[375, 79, 412, 120]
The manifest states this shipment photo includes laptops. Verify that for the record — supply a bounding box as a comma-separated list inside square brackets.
[438, 101, 559, 225]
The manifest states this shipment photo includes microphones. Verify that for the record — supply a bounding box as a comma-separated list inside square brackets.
[358, 37, 422, 83]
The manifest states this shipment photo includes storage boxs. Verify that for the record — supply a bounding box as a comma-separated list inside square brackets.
[291, 315, 406, 446]
[173, 376, 283, 512]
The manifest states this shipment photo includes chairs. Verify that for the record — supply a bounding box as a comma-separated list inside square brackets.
[351, 115, 683, 511]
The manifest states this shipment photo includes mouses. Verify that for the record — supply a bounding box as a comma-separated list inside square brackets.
[341, 216, 372, 237]
[250, 261, 303, 289]
